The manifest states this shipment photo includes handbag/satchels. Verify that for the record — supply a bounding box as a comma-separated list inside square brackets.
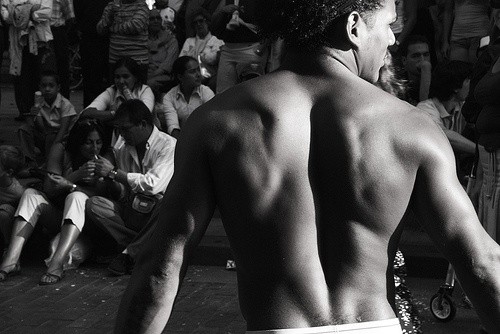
[124, 193, 156, 232]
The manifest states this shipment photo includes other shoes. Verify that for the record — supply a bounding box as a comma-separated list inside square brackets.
[14, 114, 25, 122]
[109, 253, 130, 276]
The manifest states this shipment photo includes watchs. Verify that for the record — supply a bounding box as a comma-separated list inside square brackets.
[107, 167, 119, 182]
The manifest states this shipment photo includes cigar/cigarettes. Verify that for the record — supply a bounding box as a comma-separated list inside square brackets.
[93, 152, 99, 160]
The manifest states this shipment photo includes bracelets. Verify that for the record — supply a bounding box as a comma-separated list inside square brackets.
[67, 184, 76, 192]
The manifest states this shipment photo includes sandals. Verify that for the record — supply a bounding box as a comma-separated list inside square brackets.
[0, 262, 21, 282]
[41, 267, 66, 285]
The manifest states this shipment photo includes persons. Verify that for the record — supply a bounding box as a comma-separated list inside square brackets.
[0, 0, 500, 284]
[115, 1, 500, 334]
